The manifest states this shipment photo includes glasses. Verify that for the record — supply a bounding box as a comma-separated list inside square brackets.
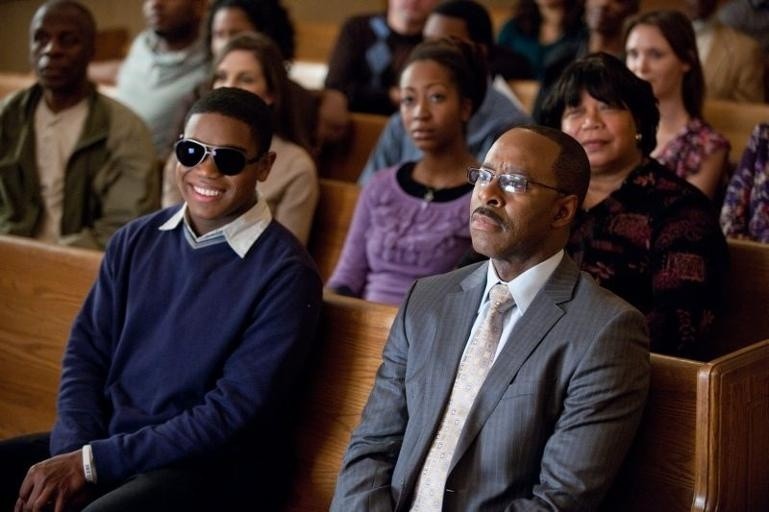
[463, 165, 566, 194]
[173, 138, 261, 175]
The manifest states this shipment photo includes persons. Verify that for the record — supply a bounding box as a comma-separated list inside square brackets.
[329, 123, 652, 512]
[1, 90, 335, 511]
[1, 0, 768, 361]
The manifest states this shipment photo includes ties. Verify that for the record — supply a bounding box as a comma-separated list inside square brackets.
[408, 284, 516, 512]
